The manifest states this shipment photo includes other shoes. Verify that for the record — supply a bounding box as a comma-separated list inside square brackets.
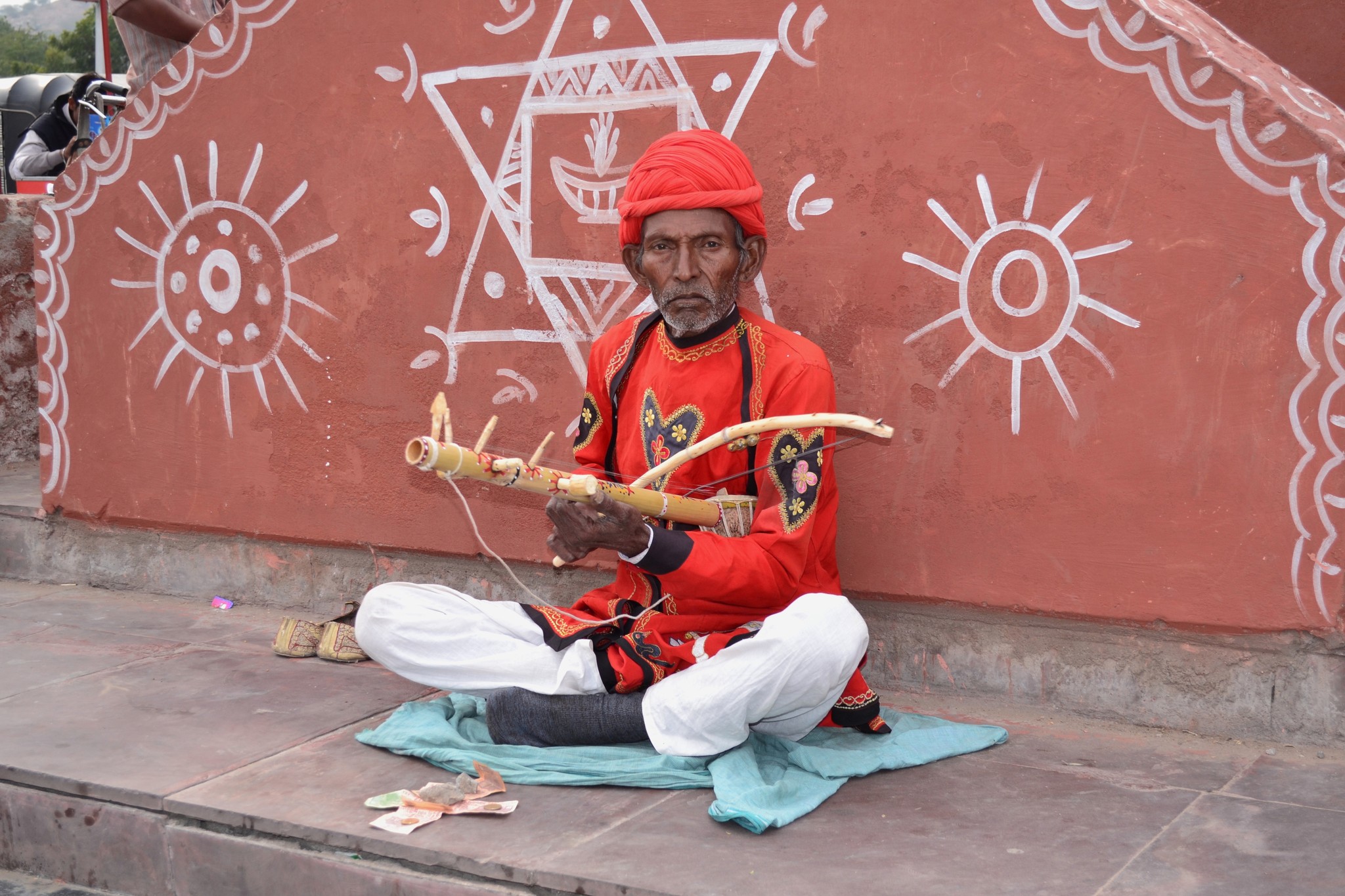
[316, 622, 369, 663]
[272, 600, 361, 659]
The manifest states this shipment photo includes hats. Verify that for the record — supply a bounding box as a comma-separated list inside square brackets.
[617, 129, 767, 248]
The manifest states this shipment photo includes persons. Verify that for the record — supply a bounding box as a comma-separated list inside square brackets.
[356, 129, 892, 756]
